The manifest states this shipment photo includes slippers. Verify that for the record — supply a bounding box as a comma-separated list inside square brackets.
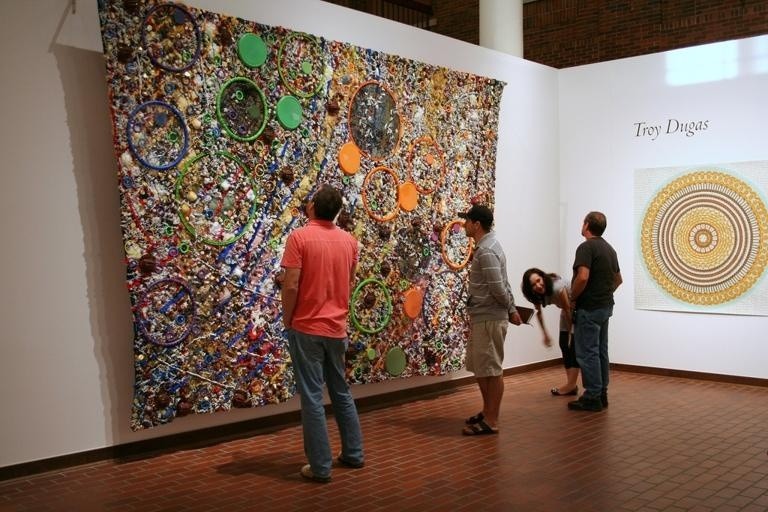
[465, 411, 483, 424]
[463, 420, 499, 436]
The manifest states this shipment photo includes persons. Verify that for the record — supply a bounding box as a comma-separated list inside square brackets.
[457, 203, 524, 437]
[566, 210, 623, 412]
[521, 267, 580, 397]
[279, 183, 365, 485]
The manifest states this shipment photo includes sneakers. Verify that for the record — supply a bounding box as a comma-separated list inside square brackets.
[336, 451, 363, 468]
[301, 464, 331, 482]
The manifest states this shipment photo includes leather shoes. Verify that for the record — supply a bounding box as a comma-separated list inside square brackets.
[551, 385, 578, 395]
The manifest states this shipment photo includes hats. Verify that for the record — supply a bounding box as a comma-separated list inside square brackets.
[458, 206, 493, 223]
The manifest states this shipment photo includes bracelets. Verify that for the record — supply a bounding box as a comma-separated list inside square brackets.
[567, 289, 576, 302]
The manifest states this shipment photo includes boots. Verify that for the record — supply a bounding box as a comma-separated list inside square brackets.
[568, 387, 608, 412]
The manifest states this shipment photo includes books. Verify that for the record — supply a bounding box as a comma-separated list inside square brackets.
[515, 304, 535, 325]
